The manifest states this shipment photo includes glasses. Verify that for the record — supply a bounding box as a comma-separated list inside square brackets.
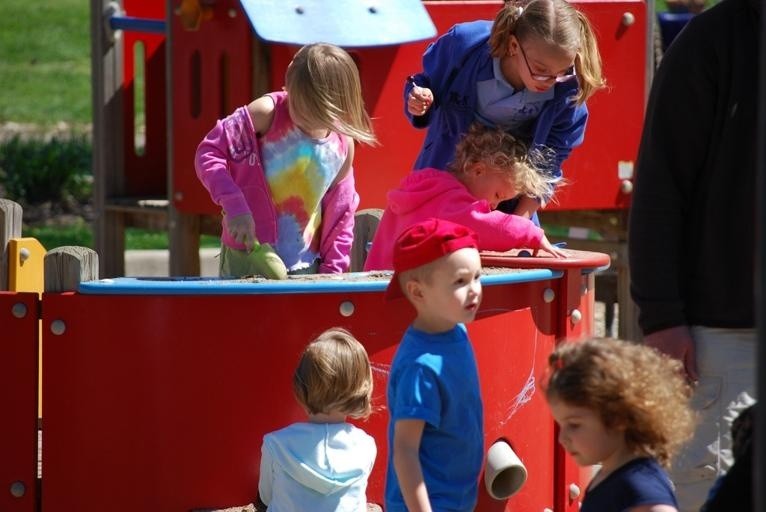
[515, 34, 576, 82]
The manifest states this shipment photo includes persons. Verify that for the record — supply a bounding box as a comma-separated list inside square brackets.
[387, 226, 485, 511]
[539, 339, 695, 512]
[629, 2, 764, 512]
[404, 1, 606, 227]
[258, 328, 378, 511]
[193, 41, 375, 279]
[363, 129, 568, 271]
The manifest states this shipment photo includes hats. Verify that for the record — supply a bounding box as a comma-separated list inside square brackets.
[384, 219, 478, 301]
